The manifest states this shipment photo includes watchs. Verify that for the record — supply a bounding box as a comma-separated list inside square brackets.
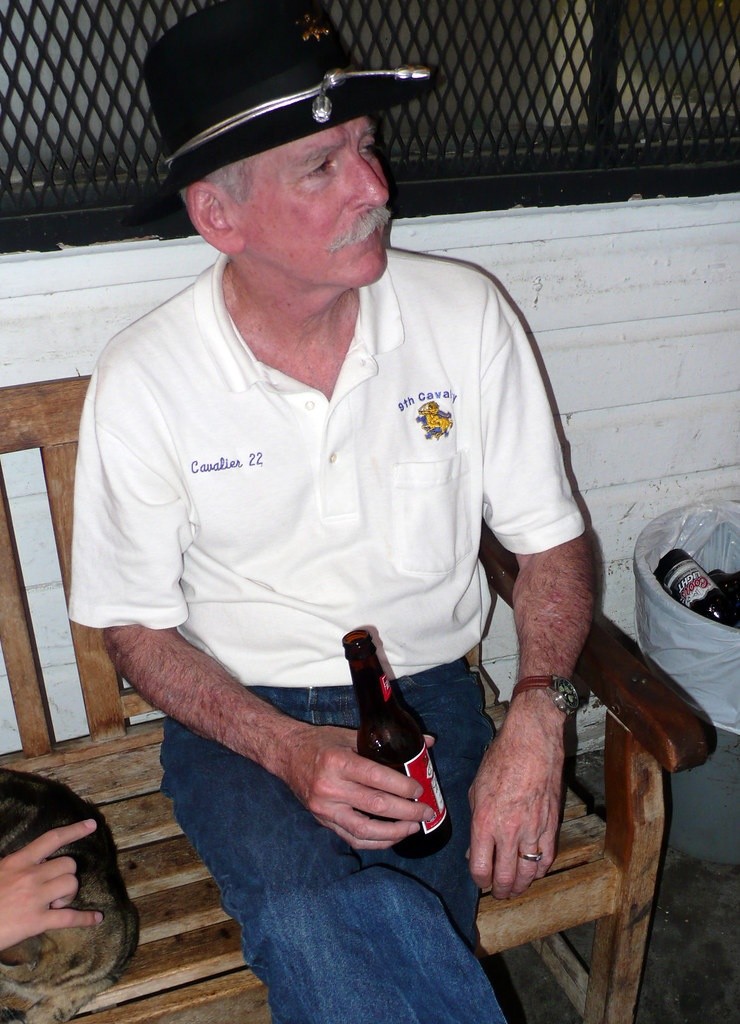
[510, 674, 579, 715]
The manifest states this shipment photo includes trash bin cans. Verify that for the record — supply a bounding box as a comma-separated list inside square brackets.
[632, 498, 740, 867]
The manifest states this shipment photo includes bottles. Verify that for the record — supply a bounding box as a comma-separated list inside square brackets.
[710, 567, 739, 594]
[339, 626, 451, 858]
[655, 549, 737, 634]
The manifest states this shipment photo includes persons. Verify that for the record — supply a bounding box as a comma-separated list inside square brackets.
[0, 819, 103, 953]
[68, 0, 595, 1024]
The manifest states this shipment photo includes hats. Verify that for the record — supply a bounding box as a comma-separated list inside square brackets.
[143, 0, 436, 186]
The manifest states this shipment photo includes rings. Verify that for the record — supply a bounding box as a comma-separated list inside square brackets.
[518, 851, 543, 862]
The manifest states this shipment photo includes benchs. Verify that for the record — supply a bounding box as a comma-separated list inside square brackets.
[1, 376, 715, 1024]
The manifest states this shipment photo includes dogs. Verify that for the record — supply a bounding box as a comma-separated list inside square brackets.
[0, 768, 141, 1024]
